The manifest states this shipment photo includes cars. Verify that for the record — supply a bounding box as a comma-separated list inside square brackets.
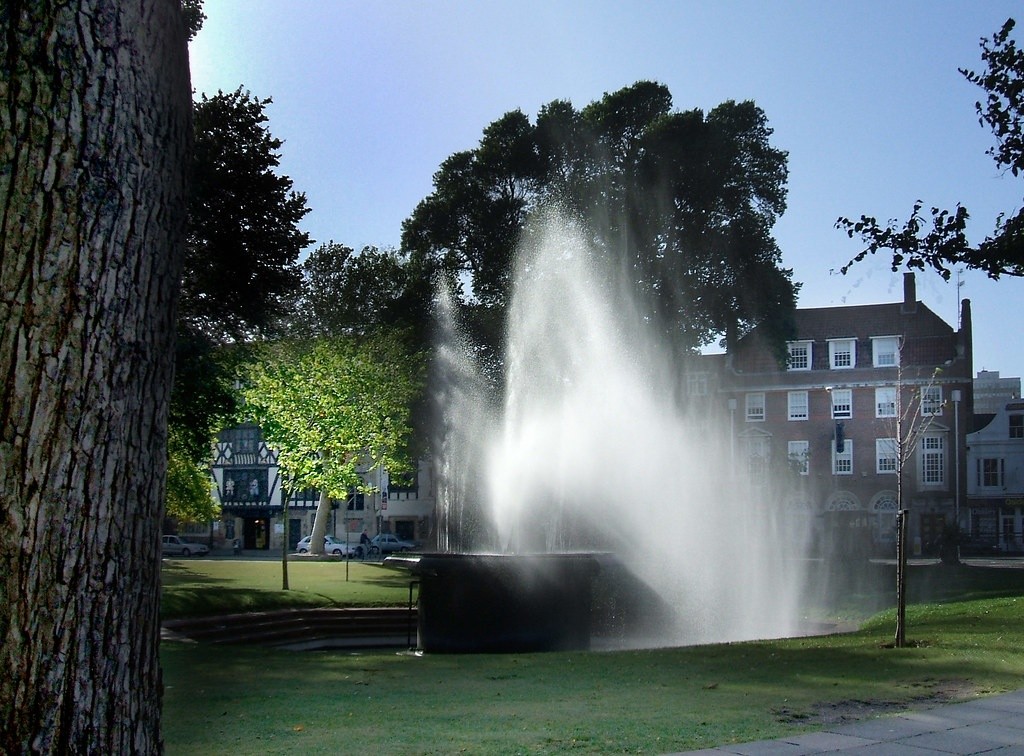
[370, 534, 414, 554]
[295, 529, 366, 558]
[162, 534, 210, 557]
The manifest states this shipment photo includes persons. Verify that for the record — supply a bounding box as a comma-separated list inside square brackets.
[231, 538, 240, 558]
[225, 477, 235, 496]
[360, 528, 373, 561]
[249, 479, 259, 499]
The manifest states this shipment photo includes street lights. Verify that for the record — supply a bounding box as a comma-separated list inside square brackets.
[728, 398, 738, 472]
[951, 389, 962, 558]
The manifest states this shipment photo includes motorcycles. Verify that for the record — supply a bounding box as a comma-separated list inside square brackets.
[232, 538, 241, 554]
